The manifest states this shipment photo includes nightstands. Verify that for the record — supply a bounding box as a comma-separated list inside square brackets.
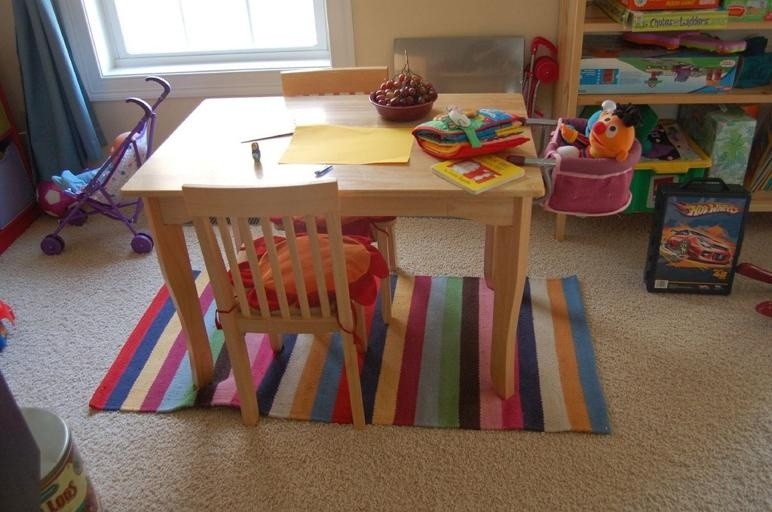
[575, 41, 740, 96]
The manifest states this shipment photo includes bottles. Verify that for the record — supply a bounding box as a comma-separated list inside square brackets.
[251, 143, 260, 162]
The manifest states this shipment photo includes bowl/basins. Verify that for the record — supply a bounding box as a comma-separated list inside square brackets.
[369, 96, 437, 122]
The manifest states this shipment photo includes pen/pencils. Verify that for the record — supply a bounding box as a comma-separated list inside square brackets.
[315, 166, 333, 174]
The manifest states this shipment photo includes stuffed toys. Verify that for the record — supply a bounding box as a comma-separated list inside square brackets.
[546, 100, 640, 167]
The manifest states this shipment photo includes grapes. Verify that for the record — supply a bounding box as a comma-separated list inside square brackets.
[375, 71, 437, 107]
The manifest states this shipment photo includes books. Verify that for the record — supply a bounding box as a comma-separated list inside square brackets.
[431, 154, 526, 196]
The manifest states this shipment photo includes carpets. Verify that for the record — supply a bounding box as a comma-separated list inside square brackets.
[89, 269, 610, 434]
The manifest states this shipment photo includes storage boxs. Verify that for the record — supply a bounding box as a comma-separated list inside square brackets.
[679, 103, 762, 188]
[642, 177, 750, 296]
[616, 118, 713, 215]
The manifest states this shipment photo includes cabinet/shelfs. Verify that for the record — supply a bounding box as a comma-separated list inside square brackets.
[551, 1, 772, 241]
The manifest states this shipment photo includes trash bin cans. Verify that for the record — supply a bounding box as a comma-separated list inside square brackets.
[18, 408, 106, 512]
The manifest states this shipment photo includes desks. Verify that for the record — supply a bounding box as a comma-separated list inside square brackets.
[118, 91, 546, 402]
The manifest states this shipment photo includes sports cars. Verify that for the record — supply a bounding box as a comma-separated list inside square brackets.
[666, 228, 732, 264]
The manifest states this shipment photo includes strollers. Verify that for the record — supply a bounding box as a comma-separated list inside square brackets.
[41, 76, 174, 255]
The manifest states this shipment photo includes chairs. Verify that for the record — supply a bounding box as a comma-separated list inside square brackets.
[181, 181, 391, 431]
[273, 68, 400, 326]
